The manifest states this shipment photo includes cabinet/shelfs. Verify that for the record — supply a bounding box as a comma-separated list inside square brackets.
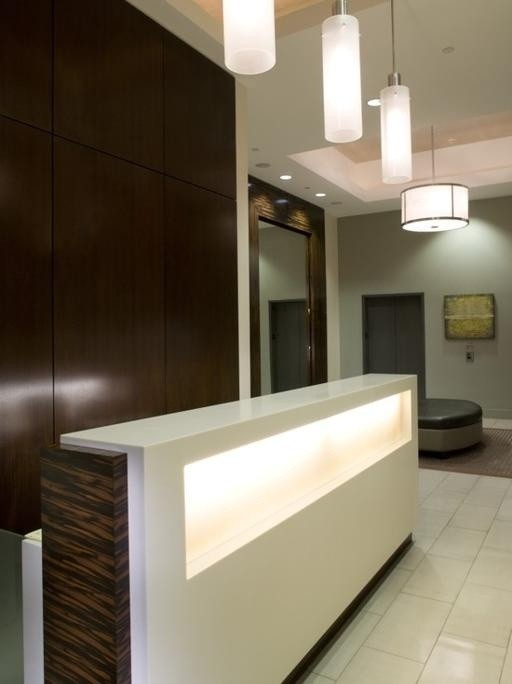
[0, 0, 239, 538]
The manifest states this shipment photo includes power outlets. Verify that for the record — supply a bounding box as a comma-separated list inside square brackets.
[464, 351, 474, 361]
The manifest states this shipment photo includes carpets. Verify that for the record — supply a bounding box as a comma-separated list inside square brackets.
[418, 427, 512, 479]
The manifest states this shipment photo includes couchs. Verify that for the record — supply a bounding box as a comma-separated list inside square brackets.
[418, 398, 484, 459]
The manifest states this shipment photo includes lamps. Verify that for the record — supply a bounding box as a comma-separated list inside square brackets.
[399, 125, 470, 234]
[379, 0, 414, 186]
[222, 0, 277, 76]
[321, 0, 363, 144]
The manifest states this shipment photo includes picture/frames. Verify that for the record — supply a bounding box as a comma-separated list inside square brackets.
[442, 293, 496, 341]
